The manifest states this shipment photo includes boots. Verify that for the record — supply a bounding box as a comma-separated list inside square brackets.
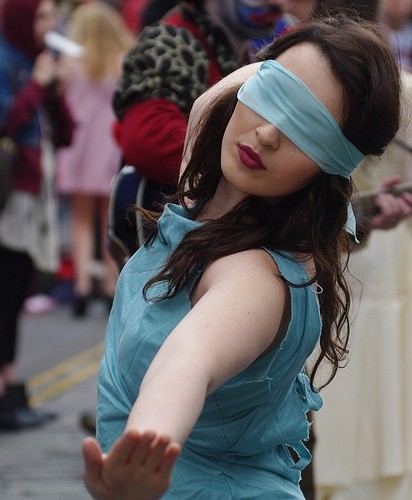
[0, 378, 58, 431]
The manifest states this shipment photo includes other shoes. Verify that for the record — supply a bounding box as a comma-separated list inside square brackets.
[71, 293, 115, 319]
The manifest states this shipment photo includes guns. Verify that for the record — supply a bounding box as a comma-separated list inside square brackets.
[340, 182, 412, 252]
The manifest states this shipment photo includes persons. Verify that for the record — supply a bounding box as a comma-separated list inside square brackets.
[80, 8, 400, 500]
[110, 0, 412, 500]
[0, 0, 412, 434]
